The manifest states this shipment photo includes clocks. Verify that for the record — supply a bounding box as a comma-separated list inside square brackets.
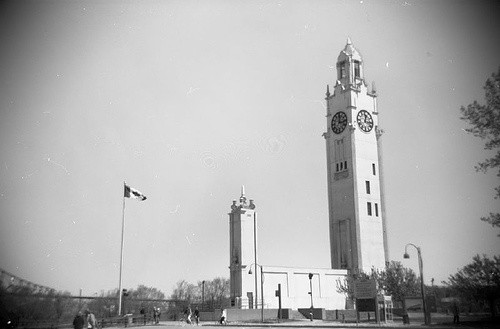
[331, 111, 348, 134]
[356, 109, 374, 132]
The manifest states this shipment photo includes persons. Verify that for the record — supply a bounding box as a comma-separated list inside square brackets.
[193, 307, 200, 324]
[72, 312, 85, 329]
[84, 309, 95, 329]
[452, 303, 460, 325]
[219, 309, 226, 324]
[154, 307, 161, 325]
[140, 306, 147, 325]
[184, 306, 192, 323]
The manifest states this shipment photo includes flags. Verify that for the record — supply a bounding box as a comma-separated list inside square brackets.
[124, 184, 147, 201]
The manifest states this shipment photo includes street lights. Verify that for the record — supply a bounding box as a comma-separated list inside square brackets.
[248, 263, 264, 323]
[403, 243, 427, 325]
